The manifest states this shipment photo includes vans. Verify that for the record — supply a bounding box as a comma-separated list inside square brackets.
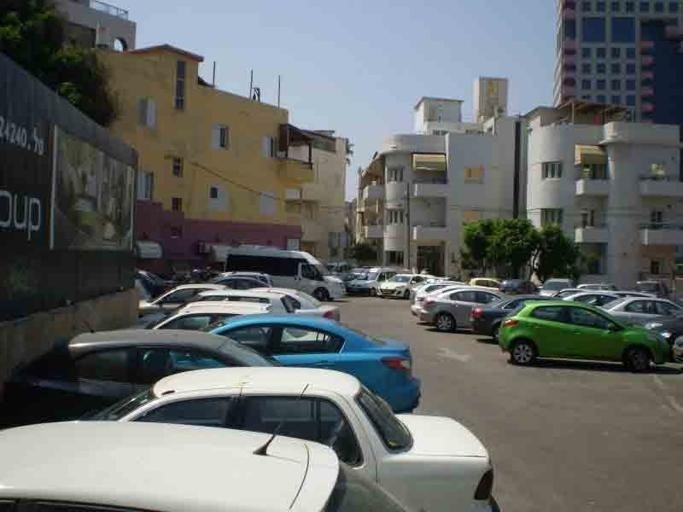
[223, 250, 346, 302]
[347, 268, 397, 297]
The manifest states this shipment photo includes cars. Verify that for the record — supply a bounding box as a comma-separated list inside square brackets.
[79, 367, 503, 512]
[142, 313, 420, 413]
[644, 308, 683, 363]
[0, 419, 410, 512]
[598, 297, 683, 330]
[499, 299, 670, 373]
[132, 264, 340, 329]
[419, 286, 509, 332]
[376, 274, 424, 300]
[0, 330, 286, 430]
[468, 295, 564, 342]
[410, 281, 467, 319]
[467, 277, 677, 306]
